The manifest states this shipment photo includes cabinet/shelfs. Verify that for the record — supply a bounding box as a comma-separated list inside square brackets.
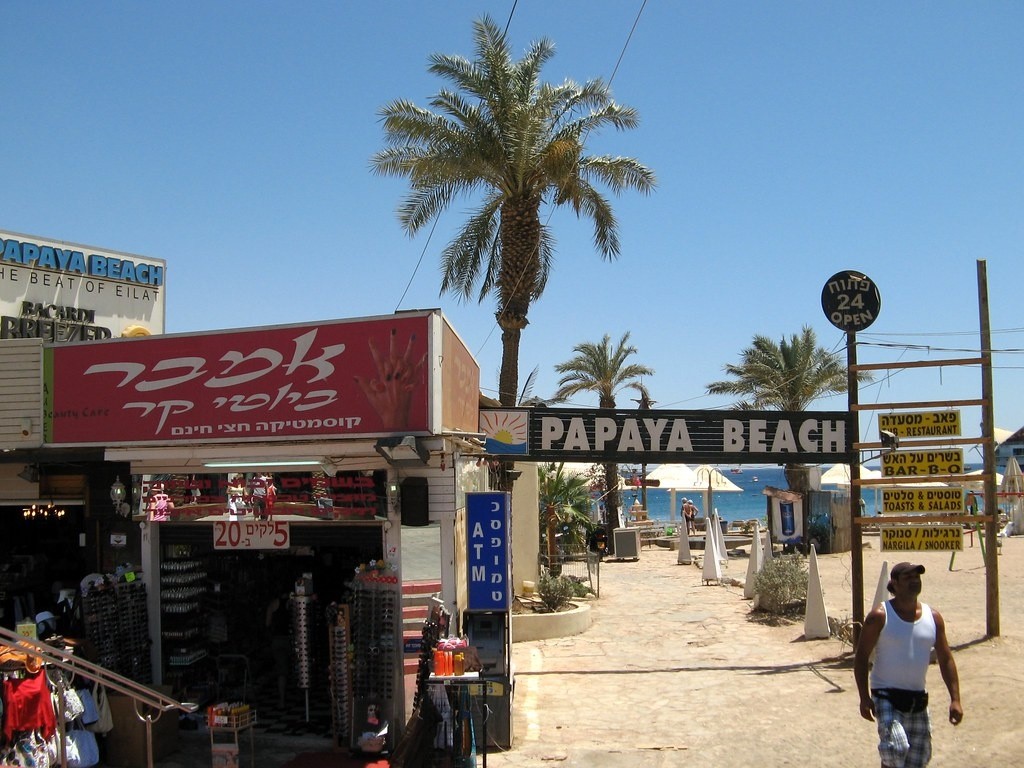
[106, 683, 180, 767]
[160, 560, 212, 671]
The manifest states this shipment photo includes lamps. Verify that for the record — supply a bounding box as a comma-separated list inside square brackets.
[110, 476, 127, 514]
[22, 504, 65, 520]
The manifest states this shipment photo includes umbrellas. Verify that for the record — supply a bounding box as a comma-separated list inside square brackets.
[638, 464, 744, 524]
[958, 456, 1024, 511]
[821, 462, 948, 514]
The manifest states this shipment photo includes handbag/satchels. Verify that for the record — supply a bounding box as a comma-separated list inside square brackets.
[0, 719, 99, 768]
[86, 664, 113, 735]
[75, 688, 99, 724]
[885, 688, 929, 713]
[49, 670, 84, 721]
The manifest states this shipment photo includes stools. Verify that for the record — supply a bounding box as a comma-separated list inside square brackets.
[211, 725, 255, 768]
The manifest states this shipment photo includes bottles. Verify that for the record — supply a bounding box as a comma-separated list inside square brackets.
[434, 650, 468, 676]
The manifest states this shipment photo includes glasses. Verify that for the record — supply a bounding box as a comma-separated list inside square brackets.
[83, 579, 154, 688]
[328, 587, 397, 735]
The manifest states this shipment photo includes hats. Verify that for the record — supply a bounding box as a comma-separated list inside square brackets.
[687, 500, 693, 503]
[79, 573, 104, 597]
[36, 611, 57, 623]
[682, 497, 687, 502]
[891, 562, 925, 574]
[56, 589, 75, 604]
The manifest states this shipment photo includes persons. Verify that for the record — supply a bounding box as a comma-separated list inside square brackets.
[969, 490, 978, 514]
[223, 473, 250, 522]
[144, 483, 174, 521]
[248, 473, 276, 520]
[687, 500, 698, 535]
[681, 497, 691, 535]
[187, 474, 202, 505]
[853, 562, 963, 768]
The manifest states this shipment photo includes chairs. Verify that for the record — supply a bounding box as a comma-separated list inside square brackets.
[695, 517, 760, 534]
